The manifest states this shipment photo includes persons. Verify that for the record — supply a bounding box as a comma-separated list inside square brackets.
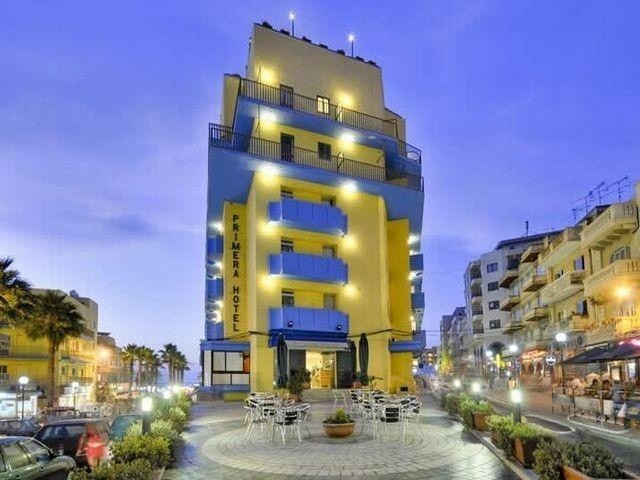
[76, 419, 113, 472]
[602, 380, 612, 397]
[588, 379, 598, 397]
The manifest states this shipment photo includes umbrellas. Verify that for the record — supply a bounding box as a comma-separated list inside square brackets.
[348, 341, 360, 384]
[356, 332, 374, 386]
[274, 335, 289, 400]
